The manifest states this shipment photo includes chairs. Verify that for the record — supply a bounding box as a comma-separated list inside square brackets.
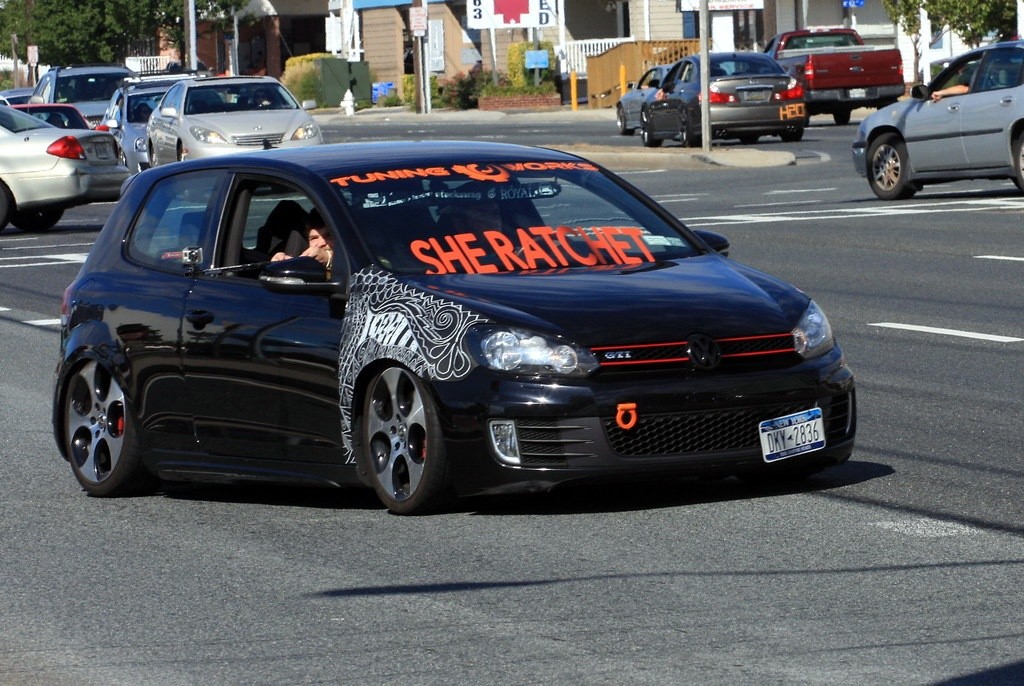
[710, 67, 726, 76]
[188, 100, 208, 113]
[256, 200, 310, 270]
[236, 96, 251, 106]
[988, 68, 1010, 90]
[180, 211, 251, 265]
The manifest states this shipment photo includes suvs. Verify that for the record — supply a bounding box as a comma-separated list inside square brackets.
[28, 64, 142, 130]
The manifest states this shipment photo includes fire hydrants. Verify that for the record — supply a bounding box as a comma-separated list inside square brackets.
[338, 88, 358, 116]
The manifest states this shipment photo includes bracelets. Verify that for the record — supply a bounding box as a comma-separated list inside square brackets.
[323, 247, 334, 269]
[259, 104, 262, 107]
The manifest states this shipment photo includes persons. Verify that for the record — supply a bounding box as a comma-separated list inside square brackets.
[254, 87, 272, 107]
[931, 57, 1012, 103]
[446, 182, 563, 256]
[268, 206, 337, 282]
[653, 77, 677, 102]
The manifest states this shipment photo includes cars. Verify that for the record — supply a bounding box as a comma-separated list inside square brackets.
[49, 139, 860, 517]
[849, 38, 1023, 201]
[616, 62, 676, 135]
[10, 104, 93, 130]
[639, 50, 808, 148]
[0, 105, 132, 235]
[146, 76, 325, 167]
[0, 87, 37, 105]
[101, 75, 194, 173]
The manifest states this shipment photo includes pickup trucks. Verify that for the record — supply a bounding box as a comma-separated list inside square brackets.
[759, 25, 907, 126]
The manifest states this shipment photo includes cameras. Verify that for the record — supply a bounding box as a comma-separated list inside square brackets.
[182, 246, 203, 265]
